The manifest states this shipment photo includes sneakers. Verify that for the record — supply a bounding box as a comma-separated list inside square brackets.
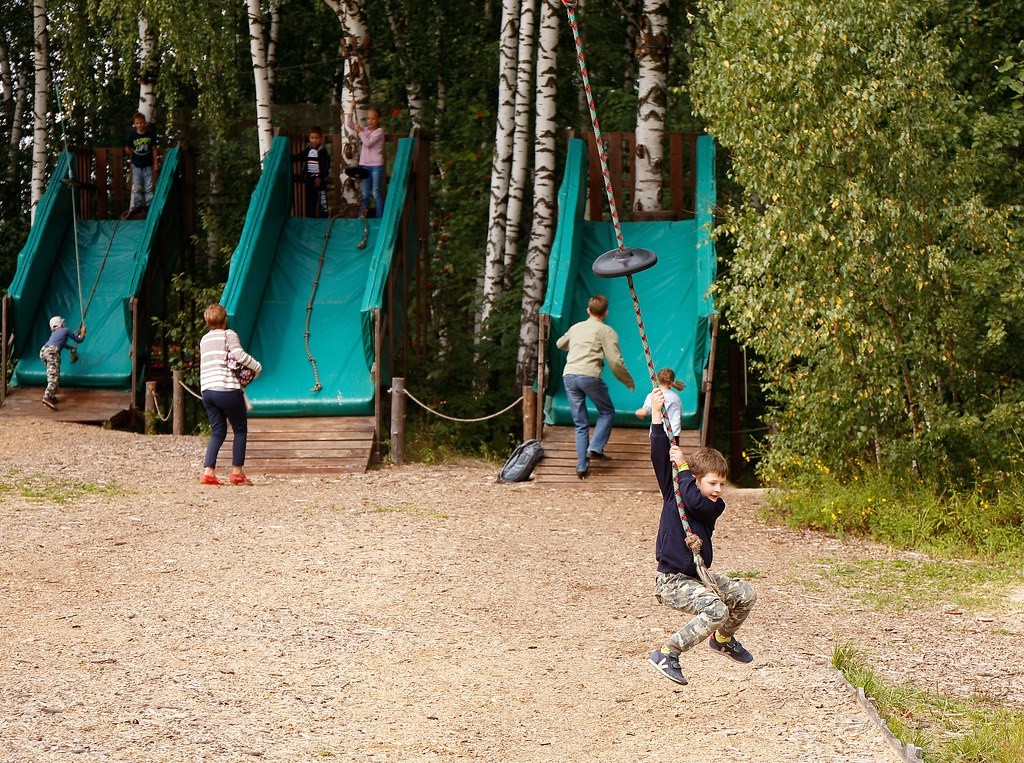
[709, 632, 754, 664]
[647, 648, 688, 685]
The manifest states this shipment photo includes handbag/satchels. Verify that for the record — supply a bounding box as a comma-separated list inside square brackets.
[223, 330, 255, 386]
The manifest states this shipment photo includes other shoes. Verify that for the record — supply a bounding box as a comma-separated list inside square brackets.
[586, 451, 610, 461]
[230, 473, 252, 486]
[576, 470, 588, 479]
[42, 396, 57, 410]
[202, 474, 224, 485]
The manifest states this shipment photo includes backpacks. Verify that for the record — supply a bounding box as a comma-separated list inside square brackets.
[498, 439, 545, 482]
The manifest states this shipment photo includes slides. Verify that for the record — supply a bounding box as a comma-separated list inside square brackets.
[6, 140, 186, 390]
[542, 131, 722, 427]
[217, 129, 422, 414]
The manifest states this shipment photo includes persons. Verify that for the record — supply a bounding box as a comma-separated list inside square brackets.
[124, 112, 160, 219]
[198, 302, 263, 486]
[38, 315, 88, 411]
[554, 294, 637, 481]
[635, 368, 683, 503]
[648, 399, 757, 686]
[347, 99, 383, 217]
[291, 126, 331, 218]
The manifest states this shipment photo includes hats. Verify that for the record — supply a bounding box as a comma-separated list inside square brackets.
[50, 316, 65, 331]
